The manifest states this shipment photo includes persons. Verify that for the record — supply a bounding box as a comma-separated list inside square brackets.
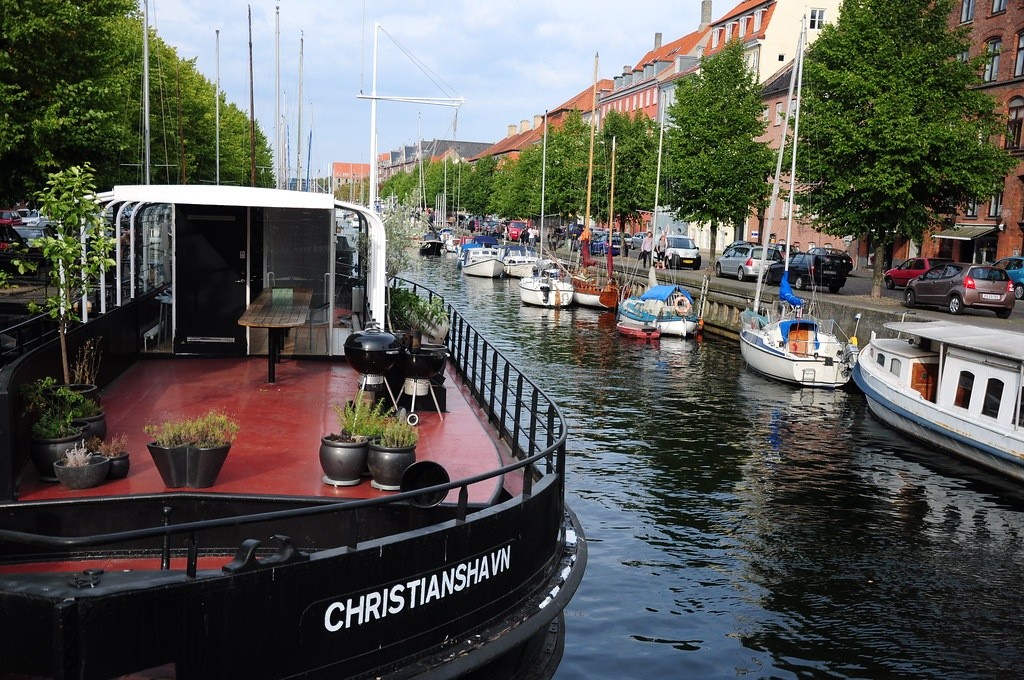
[656, 236, 666, 269]
[520, 226, 539, 247]
[469, 219, 475, 233]
[642, 231, 653, 269]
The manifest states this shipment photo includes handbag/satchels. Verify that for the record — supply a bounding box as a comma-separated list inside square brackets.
[654, 240, 660, 252]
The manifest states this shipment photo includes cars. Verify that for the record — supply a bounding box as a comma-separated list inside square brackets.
[714, 244, 785, 282]
[732, 240, 761, 257]
[767, 242, 795, 260]
[653, 235, 701, 271]
[903, 261, 1016, 319]
[765, 251, 851, 293]
[805, 246, 854, 273]
[593, 225, 648, 254]
[884, 257, 956, 290]
[978, 257, 1024, 299]
[0, 206, 116, 275]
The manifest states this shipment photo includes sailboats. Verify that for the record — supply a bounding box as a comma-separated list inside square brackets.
[421, 48, 630, 311]
[611, 88, 702, 339]
[738, 11, 862, 388]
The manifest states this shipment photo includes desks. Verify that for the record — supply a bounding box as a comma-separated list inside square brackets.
[239, 286, 315, 382]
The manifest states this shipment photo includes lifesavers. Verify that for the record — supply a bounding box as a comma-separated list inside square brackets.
[457, 259, 461, 268]
[674, 296, 690, 313]
[850, 337, 857, 347]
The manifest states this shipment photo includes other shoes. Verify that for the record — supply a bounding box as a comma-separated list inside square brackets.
[656, 266, 660, 270]
[662, 267, 666, 270]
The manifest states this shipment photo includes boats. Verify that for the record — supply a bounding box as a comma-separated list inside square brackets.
[614, 319, 663, 341]
[0, 181, 592, 680]
[849, 320, 1024, 483]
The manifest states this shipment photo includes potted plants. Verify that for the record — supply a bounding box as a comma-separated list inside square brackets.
[1, 158, 131, 490]
[317, 378, 420, 488]
[144, 408, 236, 488]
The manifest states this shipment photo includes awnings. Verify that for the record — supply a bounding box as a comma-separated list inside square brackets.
[931, 227, 996, 240]
